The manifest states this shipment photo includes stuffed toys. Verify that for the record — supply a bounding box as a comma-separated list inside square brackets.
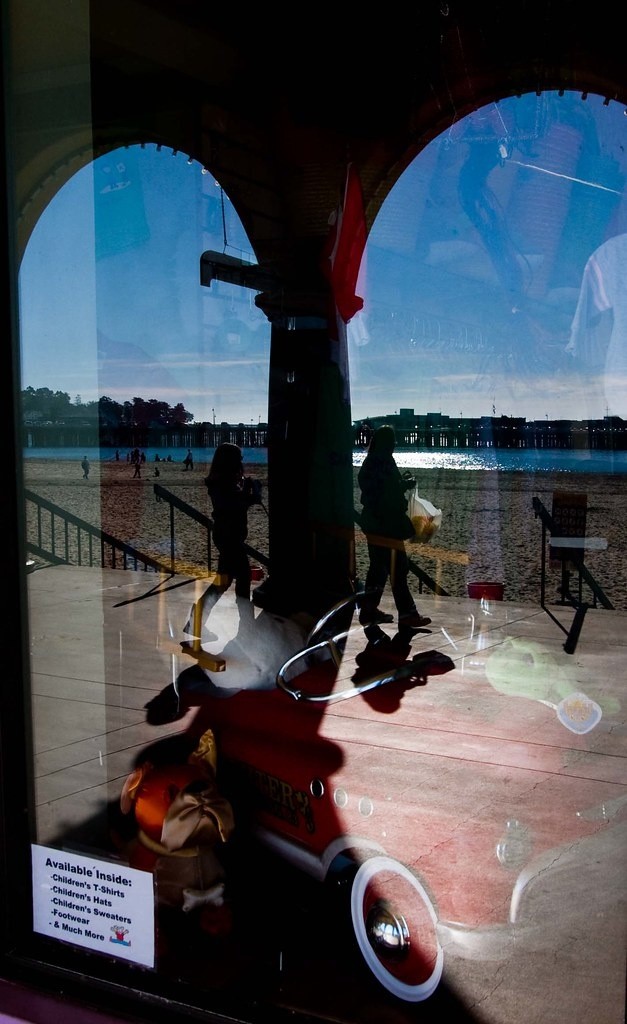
[120, 728, 234, 912]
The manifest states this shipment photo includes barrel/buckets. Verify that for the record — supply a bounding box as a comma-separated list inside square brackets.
[467, 582, 505, 600]
[250, 565, 262, 581]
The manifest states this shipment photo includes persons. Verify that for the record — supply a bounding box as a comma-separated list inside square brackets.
[115, 450, 120, 461]
[155, 454, 160, 461]
[81, 456, 91, 479]
[166, 455, 171, 462]
[182, 443, 262, 645]
[358, 425, 432, 628]
[127, 448, 146, 465]
[183, 449, 193, 471]
[133, 459, 141, 478]
[154, 467, 160, 477]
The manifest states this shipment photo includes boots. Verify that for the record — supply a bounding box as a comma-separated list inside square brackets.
[237, 601, 257, 635]
[182, 603, 217, 642]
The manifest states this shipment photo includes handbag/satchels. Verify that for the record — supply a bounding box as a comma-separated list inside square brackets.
[406, 477, 442, 542]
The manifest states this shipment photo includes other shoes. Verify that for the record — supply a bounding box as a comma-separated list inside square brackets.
[359, 609, 393, 625]
[398, 614, 431, 630]
